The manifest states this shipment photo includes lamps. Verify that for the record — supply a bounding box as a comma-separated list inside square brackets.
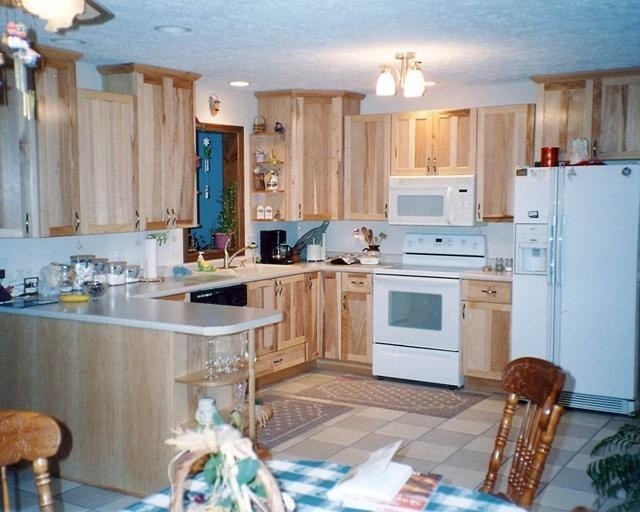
[19, 0, 112, 34]
[372, 51, 429, 101]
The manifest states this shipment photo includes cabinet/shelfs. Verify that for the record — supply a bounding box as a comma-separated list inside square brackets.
[254, 88, 302, 223]
[244, 274, 322, 379]
[478, 104, 535, 219]
[322, 270, 373, 366]
[290, 89, 358, 221]
[346, 112, 391, 222]
[391, 108, 477, 175]
[0, 42, 34, 238]
[78, 88, 144, 231]
[461, 278, 512, 382]
[101, 61, 203, 229]
[529, 66, 639, 161]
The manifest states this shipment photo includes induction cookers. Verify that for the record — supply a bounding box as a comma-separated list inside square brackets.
[374, 254, 486, 278]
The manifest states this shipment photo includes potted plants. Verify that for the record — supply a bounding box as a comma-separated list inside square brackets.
[209, 178, 236, 249]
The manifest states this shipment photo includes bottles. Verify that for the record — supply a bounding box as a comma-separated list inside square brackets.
[203, 330, 257, 381]
[505, 258, 513, 272]
[49, 255, 139, 301]
[496, 257, 503, 272]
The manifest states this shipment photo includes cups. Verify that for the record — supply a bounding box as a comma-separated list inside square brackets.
[542, 147, 559, 167]
[234, 380, 247, 412]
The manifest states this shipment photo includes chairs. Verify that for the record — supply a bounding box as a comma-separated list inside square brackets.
[0, 408, 67, 510]
[479, 357, 566, 511]
[29, 44, 82, 237]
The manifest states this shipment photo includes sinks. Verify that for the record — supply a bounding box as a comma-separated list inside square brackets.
[232, 263, 303, 275]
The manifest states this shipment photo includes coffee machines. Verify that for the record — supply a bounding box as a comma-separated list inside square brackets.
[260, 230, 294, 264]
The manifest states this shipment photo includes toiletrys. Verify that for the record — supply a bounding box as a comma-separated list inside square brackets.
[196, 251, 206, 272]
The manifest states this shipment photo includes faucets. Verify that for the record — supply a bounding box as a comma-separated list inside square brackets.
[223, 238, 258, 268]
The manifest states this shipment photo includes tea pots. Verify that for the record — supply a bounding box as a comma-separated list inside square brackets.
[363, 245, 381, 257]
[195, 399, 218, 425]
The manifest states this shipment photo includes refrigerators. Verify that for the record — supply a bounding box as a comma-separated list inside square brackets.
[510, 165, 640, 418]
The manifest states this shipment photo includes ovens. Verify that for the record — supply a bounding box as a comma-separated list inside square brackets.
[372, 275, 464, 390]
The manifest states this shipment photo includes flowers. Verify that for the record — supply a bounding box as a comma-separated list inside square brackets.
[163, 419, 295, 512]
[202, 136, 213, 158]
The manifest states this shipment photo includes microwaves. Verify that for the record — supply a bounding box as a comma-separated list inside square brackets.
[389, 175, 476, 226]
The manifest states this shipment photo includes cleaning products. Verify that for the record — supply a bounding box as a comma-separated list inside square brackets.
[256, 204, 265, 220]
[264, 205, 273, 220]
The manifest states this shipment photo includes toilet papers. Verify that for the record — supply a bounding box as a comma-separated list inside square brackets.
[142, 238, 158, 279]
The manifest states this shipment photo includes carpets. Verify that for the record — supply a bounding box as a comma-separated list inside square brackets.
[235, 389, 355, 453]
[295, 375, 490, 420]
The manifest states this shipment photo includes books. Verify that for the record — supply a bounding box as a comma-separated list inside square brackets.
[342, 471, 444, 512]
[327, 439, 413, 504]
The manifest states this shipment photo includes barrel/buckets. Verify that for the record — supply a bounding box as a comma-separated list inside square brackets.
[253, 113, 267, 134]
